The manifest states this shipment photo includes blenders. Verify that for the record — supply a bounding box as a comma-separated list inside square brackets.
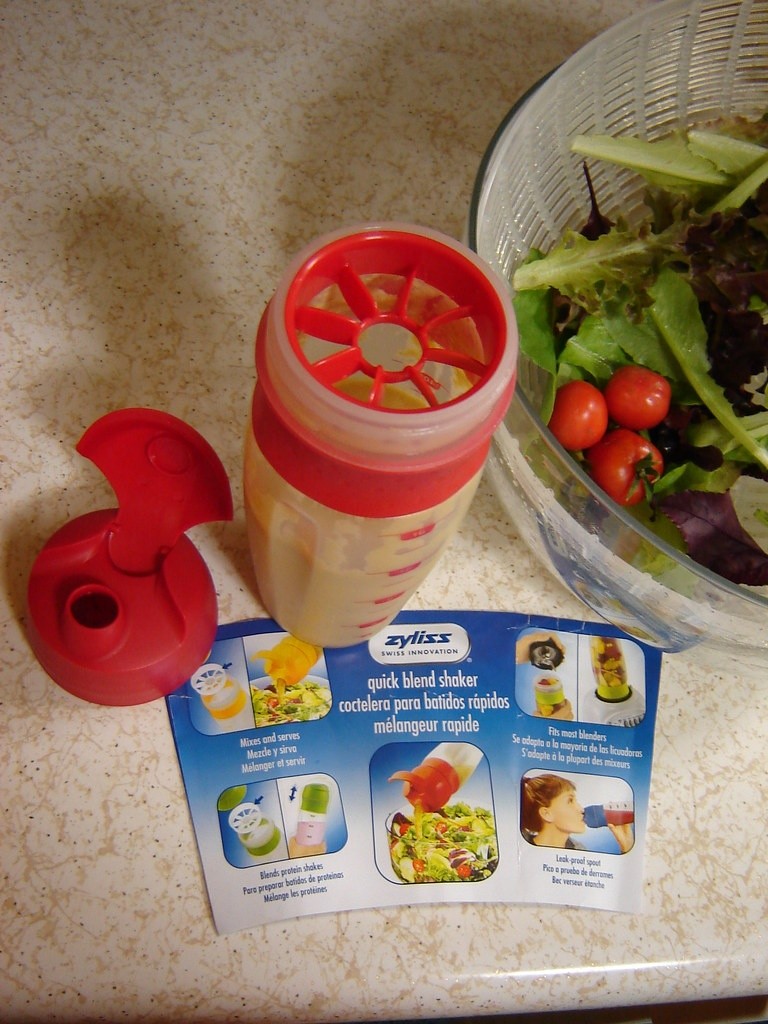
[581, 634, 646, 727]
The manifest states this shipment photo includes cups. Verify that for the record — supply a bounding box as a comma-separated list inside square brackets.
[243, 219, 517, 651]
[533, 674, 565, 717]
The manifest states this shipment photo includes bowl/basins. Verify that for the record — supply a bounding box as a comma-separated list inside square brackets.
[385, 798, 498, 883]
[460, 5, 768, 656]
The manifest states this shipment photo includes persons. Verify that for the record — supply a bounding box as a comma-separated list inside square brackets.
[518, 774, 634, 856]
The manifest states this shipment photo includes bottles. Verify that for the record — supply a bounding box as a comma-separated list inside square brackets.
[389, 740, 483, 813]
[237, 813, 287, 864]
[201, 673, 255, 732]
[251, 635, 323, 688]
[295, 782, 330, 846]
[583, 800, 633, 828]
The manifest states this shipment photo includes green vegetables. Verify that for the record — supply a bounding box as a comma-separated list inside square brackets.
[249, 677, 332, 727]
[498, 103, 768, 607]
[387, 801, 499, 883]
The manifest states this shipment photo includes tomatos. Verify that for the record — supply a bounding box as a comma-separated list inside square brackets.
[548, 380, 609, 451]
[585, 427, 665, 521]
[435, 822, 447, 833]
[603, 365, 672, 431]
[458, 865, 472, 877]
[399, 823, 411, 835]
[413, 859, 426, 872]
